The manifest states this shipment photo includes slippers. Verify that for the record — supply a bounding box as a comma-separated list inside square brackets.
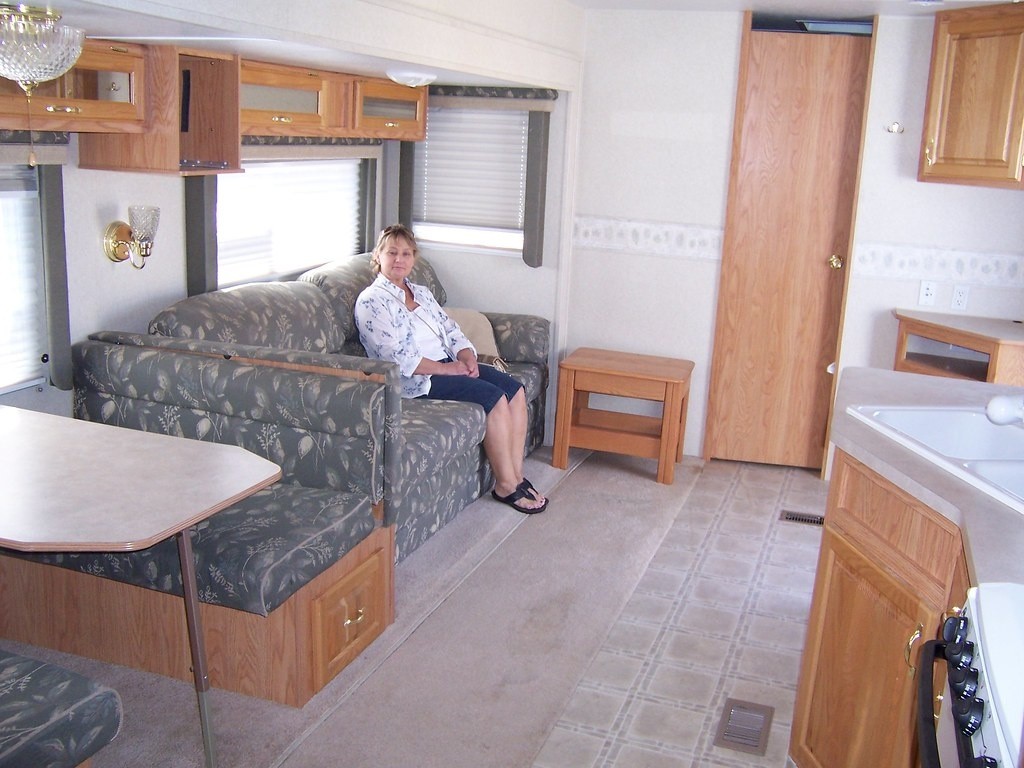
[520, 478, 550, 505]
[492, 485, 547, 514]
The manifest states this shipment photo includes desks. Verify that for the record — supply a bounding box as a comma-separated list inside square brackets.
[0, 403, 281, 768]
[890, 307, 1024, 387]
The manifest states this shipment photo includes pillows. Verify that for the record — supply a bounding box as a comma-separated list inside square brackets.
[439, 306, 500, 358]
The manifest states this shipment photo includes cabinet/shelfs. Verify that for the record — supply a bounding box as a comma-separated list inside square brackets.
[0, 40, 428, 177]
[916, 1, 1024, 193]
[789, 447, 970, 768]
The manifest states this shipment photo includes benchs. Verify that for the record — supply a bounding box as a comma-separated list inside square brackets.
[0, 650, 123, 768]
[38, 331, 399, 711]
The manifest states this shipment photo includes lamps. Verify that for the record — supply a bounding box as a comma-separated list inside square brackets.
[0, 2, 87, 167]
[104, 206, 161, 269]
[386, 71, 439, 88]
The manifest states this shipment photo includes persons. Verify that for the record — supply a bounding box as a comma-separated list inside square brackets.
[354, 223, 549, 514]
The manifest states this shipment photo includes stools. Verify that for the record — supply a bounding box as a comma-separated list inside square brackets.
[552, 347, 695, 485]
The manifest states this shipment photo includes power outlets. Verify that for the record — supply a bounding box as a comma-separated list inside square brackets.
[917, 279, 937, 308]
[950, 285, 969, 311]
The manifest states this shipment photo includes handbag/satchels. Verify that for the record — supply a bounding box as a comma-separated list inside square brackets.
[476, 353, 508, 375]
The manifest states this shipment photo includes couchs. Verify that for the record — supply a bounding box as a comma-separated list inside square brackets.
[147, 251, 553, 569]
